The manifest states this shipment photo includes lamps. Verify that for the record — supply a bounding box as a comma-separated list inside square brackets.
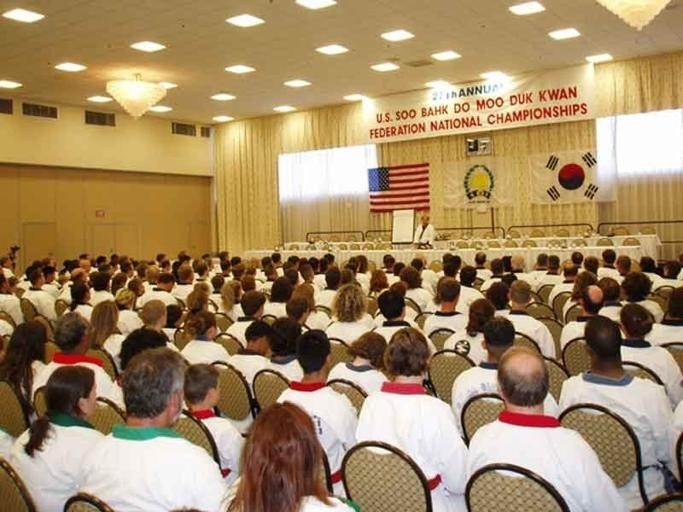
[106, 73, 167, 119]
[598, 0, 671, 32]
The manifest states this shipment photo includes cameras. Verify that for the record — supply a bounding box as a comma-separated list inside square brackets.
[10, 245, 20, 260]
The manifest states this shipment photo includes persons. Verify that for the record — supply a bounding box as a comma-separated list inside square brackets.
[413, 213, 436, 247]
[13, 366, 107, 512]
[86, 351, 224, 512]
[463, 344, 627, 512]
[0, 249, 683, 438]
[606, 227, 613, 235]
[562, 318, 669, 512]
[278, 328, 356, 499]
[665, 399, 683, 483]
[179, 365, 244, 470]
[584, 222, 595, 237]
[354, 328, 468, 512]
[217, 400, 350, 512]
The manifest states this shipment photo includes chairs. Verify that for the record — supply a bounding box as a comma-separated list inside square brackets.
[64, 493, 115, 511]
[272, 226, 656, 250]
[319, 448, 333, 493]
[340, 440, 433, 512]
[465, 463, 570, 512]
[0, 457, 37, 512]
[1, 261, 683, 460]
[647, 491, 683, 512]
[675, 432, 683, 481]
[557, 403, 648, 512]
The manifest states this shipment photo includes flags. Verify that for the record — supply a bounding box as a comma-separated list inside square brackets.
[367, 162, 430, 212]
[528, 149, 616, 204]
[444, 157, 513, 211]
